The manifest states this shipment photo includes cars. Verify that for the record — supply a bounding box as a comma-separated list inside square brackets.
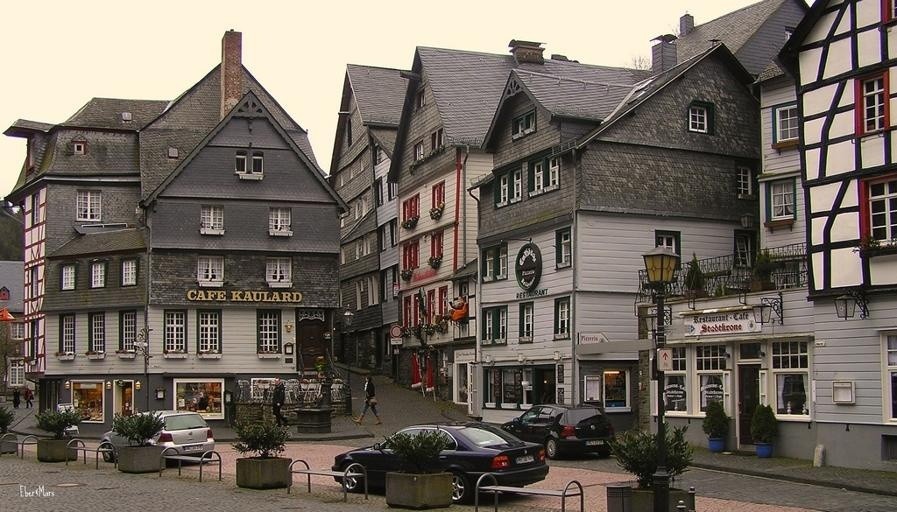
[99, 410, 214, 462]
[499, 404, 614, 459]
[331, 420, 549, 504]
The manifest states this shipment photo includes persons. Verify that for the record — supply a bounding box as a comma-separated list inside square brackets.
[24, 389, 34, 408]
[352, 376, 382, 426]
[272, 378, 287, 427]
[12, 386, 20, 408]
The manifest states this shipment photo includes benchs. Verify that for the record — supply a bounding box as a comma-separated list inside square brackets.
[63, 438, 118, 471]
[0, 432, 38, 462]
[475, 472, 584, 511]
[286, 458, 368, 503]
[155, 446, 221, 482]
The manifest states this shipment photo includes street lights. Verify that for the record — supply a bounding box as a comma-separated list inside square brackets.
[133, 325, 153, 410]
[343, 304, 354, 415]
[640, 243, 684, 511]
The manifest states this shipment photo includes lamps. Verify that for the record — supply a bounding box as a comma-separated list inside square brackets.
[830, 288, 871, 320]
[63, 381, 71, 391]
[134, 379, 142, 392]
[105, 379, 112, 391]
[752, 291, 785, 328]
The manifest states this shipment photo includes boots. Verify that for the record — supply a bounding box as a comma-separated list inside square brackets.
[374, 416, 383, 425]
[353, 415, 363, 425]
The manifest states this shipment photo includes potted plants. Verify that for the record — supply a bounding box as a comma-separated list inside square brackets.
[746, 402, 779, 457]
[698, 398, 730, 452]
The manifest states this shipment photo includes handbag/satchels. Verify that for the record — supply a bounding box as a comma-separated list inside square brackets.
[368, 398, 378, 406]
